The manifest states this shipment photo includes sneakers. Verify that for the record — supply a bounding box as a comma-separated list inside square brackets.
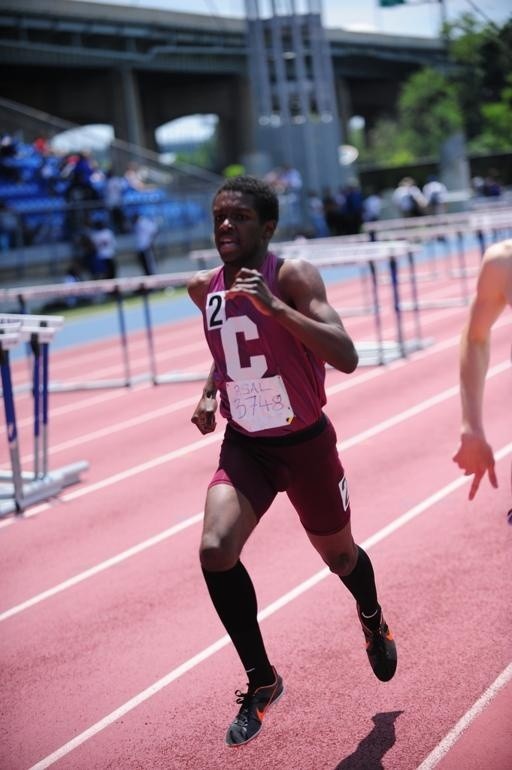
[225, 665, 284, 746]
[355, 600, 398, 682]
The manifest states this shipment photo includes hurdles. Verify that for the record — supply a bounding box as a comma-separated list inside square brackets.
[0, 270, 217, 393]
[363, 203, 512, 313]
[0, 313, 89, 513]
[189, 241, 437, 367]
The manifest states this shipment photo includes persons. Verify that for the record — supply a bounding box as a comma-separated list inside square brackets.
[450, 238, 510, 508]
[266, 164, 446, 234]
[1, 138, 157, 286]
[477, 172, 502, 197]
[185, 174, 397, 748]
